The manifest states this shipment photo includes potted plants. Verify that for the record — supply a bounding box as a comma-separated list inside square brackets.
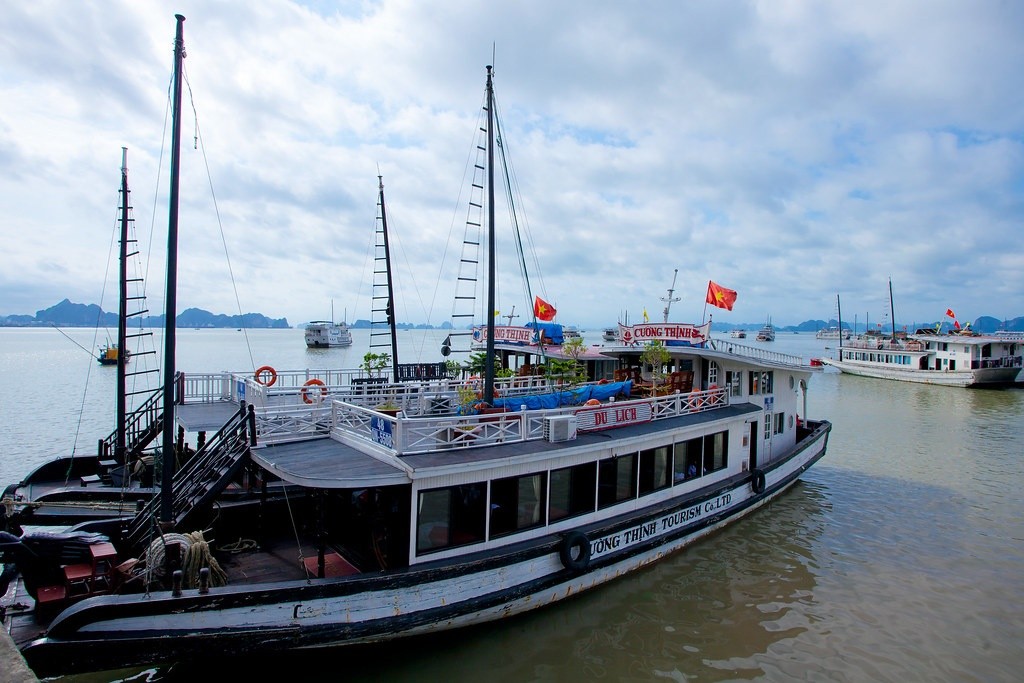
[452, 385, 482, 445]
[376, 399, 403, 425]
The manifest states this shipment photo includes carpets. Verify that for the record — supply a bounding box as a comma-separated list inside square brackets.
[304, 552, 360, 578]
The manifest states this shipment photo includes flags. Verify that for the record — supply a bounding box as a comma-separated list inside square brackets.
[706, 280, 737, 311]
[954, 321, 961, 329]
[495, 311, 500, 316]
[644, 310, 648, 320]
[946, 309, 955, 318]
[534, 296, 557, 321]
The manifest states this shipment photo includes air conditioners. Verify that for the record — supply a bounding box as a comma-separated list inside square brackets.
[543, 415, 578, 443]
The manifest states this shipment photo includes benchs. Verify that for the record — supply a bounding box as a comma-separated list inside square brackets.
[515, 363, 543, 376]
[614, 367, 694, 397]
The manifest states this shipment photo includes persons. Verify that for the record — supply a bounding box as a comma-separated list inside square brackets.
[686, 460, 707, 479]
[620, 373, 626, 382]
[417, 366, 421, 379]
[520, 364, 546, 376]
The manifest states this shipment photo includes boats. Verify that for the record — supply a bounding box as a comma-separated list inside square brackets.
[810, 275, 1024, 388]
[304, 320, 354, 348]
[815, 325, 853, 340]
[601, 326, 622, 341]
[0, 10, 833, 674]
[95, 337, 132, 363]
[559, 325, 584, 345]
[756, 316, 776, 341]
[730, 330, 747, 339]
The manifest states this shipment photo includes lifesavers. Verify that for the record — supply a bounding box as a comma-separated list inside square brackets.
[464, 376, 480, 389]
[597, 379, 610, 384]
[583, 399, 602, 406]
[301, 379, 327, 403]
[878, 344, 883, 350]
[560, 531, 591, 573]
[477, 387, 499, 398]
[751, 469, 765, 494]
[255, 365, 277, 387]
[688, 389, 702, 411]
